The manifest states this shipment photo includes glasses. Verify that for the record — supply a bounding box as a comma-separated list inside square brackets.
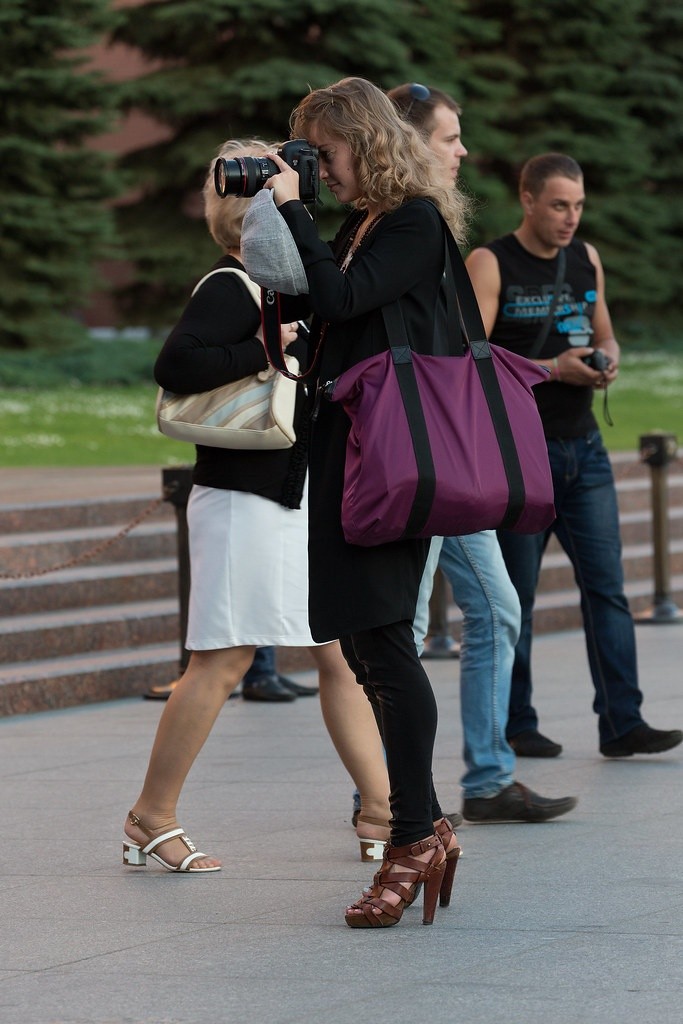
[403, 81, 428, 120]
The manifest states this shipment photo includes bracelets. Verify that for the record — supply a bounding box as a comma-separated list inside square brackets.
[554, 357, 561, 382]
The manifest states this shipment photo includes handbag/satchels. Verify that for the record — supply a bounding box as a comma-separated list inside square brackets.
[156, 268, 299, 450]
[327, 198, 558, 547]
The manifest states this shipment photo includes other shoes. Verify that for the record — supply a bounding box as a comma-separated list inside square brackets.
[506, 731, 563, 758]
[276, 673, 321, 696]
[241, 681, 294, 703]
[423, 636, 460, 660]
[598, 721, 683, 758]
[460, 779, 575, 823]
[142, 681, 180, 702]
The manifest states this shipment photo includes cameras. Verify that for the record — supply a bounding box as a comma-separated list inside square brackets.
[214, 139, 320, 205]
[584, 351, 610, 371]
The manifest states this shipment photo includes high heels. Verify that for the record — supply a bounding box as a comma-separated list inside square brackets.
[354, 812, 392, 861]
[404, 818, 461, 907]
[344, 828, 446, 929]
[121, 812, 221, 873]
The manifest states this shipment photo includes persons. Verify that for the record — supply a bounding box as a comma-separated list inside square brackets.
[264, 77, 468, 929]
[464, 152, 683, 758]
[353, 84, 578, 829]
[241, 645, 320, 703]
[123, 140, 463, 873]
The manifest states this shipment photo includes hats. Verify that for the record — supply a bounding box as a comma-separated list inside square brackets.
[240, 187, 310, 297]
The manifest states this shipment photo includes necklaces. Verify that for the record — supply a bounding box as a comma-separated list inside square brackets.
[337, 208, 386, 278]
